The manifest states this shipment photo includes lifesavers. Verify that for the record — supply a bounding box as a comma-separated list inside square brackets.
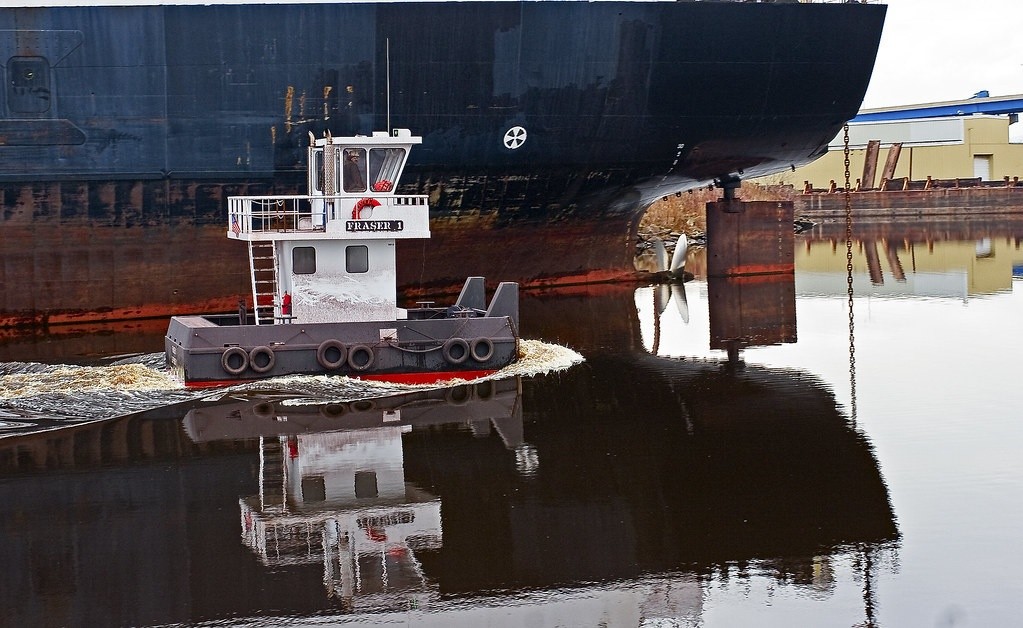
[318, 403, 350, 421]
[351, 197, 382, 220]
[249, 345, 275, 373]
[221, 346, 249, 376]
[445, 384, 473, 407]
[442, 337, 471, 365]
[470, 336, 495, 363]
[316, 339, 347, 370]
[252, 402, 275, 420]
[348, 344, 374, 372]
[349, 400, 377, 415]
[473, 380, 497, 408]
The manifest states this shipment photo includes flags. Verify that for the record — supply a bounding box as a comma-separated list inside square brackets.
[232, 216, 240, 238]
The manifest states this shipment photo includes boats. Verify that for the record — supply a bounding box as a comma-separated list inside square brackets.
[0, 2, 888, 334]
[181, 377, 526, 612]
[162, 37, 519, 391]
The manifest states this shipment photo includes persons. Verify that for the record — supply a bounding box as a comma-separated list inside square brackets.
[343, 152, 366, 193]
